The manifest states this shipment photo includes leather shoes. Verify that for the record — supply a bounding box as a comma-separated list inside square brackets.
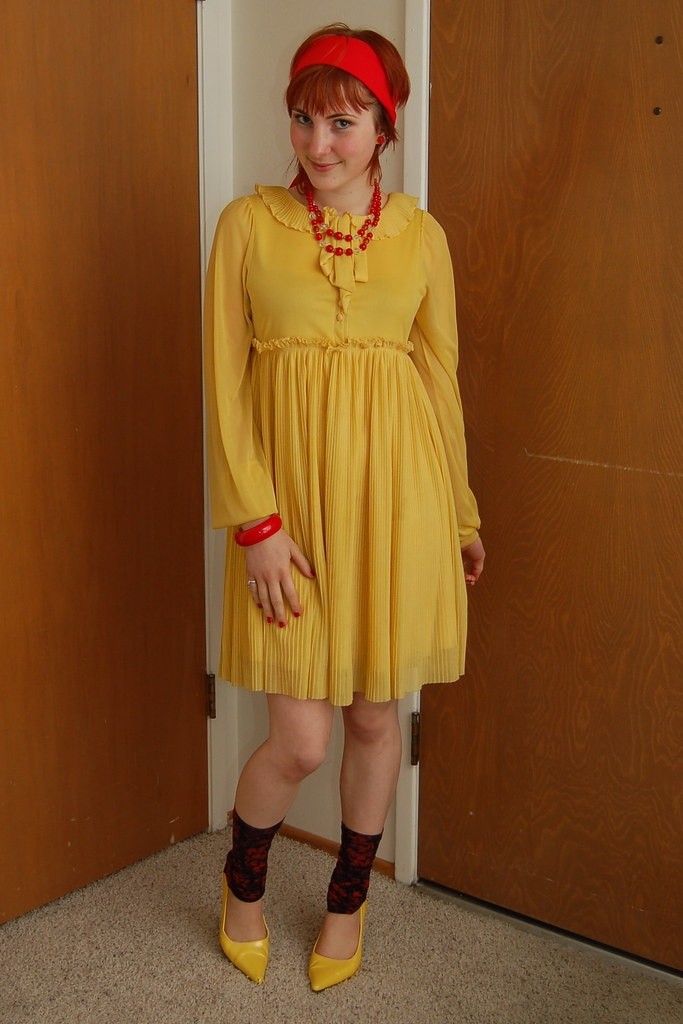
[219, 871, 271, 984]
[307, 899, 366, 992]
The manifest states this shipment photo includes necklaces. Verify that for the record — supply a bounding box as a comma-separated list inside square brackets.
[305, 178, 382, 256]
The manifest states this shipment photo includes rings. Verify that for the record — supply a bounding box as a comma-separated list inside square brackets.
[248, 580, 256, 584]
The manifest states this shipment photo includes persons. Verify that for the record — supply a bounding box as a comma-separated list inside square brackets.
[207, 24, 485, 995]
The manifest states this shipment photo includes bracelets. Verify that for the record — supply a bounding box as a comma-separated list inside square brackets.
[235, 514, 282, 547]
